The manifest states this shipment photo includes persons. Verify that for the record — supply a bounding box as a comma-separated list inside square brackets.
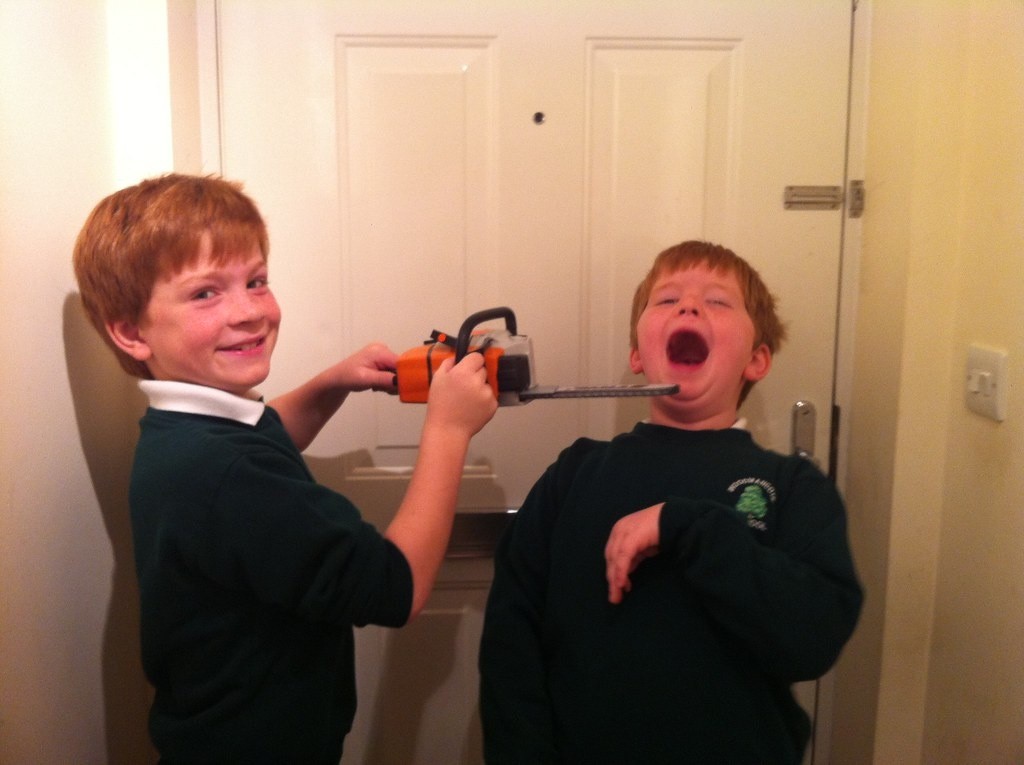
[74, 173, 498, 765]
[479, 241, 865, 765]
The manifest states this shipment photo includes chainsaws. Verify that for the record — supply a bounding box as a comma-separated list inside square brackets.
[392, 306, 681, 407]
